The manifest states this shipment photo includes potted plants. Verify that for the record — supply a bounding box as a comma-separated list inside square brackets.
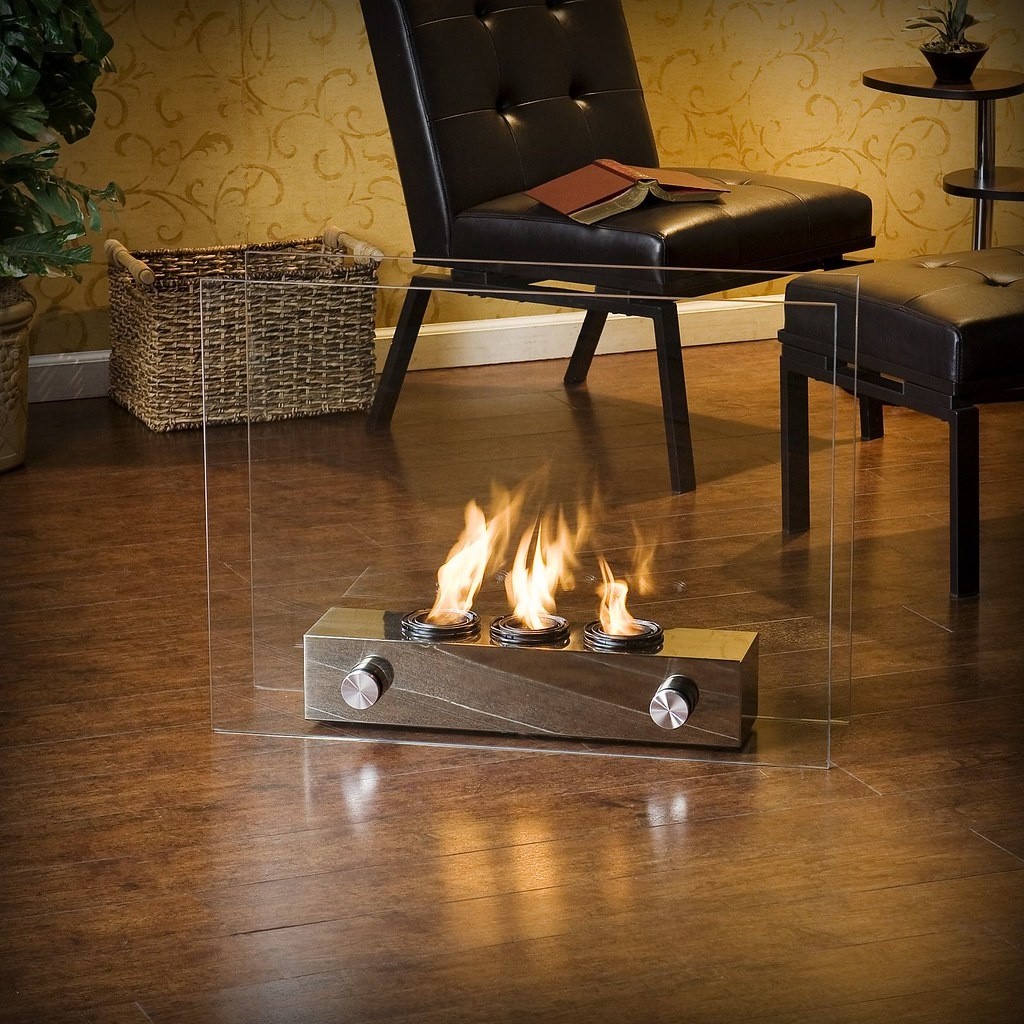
[905, 1, 989, 83]
[1, 0, 125, 476]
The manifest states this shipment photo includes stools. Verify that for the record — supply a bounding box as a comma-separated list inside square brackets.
[774, 240, 1023, 597]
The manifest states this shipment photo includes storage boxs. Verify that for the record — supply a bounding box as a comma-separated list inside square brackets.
[101, 228, 389, 434]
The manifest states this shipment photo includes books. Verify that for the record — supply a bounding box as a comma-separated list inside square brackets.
[522, 156, 732, 229]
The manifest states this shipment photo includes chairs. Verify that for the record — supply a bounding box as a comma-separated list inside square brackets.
[342, 1, 883, 500]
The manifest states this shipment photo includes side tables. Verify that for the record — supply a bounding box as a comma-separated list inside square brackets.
[859, 64, 1023, 252]
